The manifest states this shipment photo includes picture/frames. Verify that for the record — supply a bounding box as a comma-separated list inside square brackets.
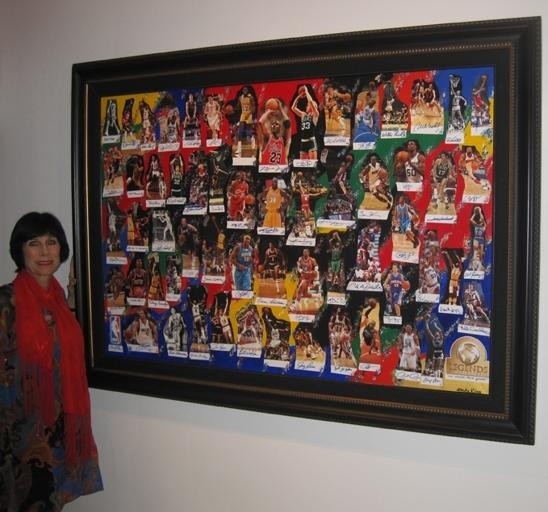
[65, 15, 541, 447]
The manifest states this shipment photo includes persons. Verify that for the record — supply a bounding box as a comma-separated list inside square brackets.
[99, 67, 493, 385]
[0, 211, 77, 512]
